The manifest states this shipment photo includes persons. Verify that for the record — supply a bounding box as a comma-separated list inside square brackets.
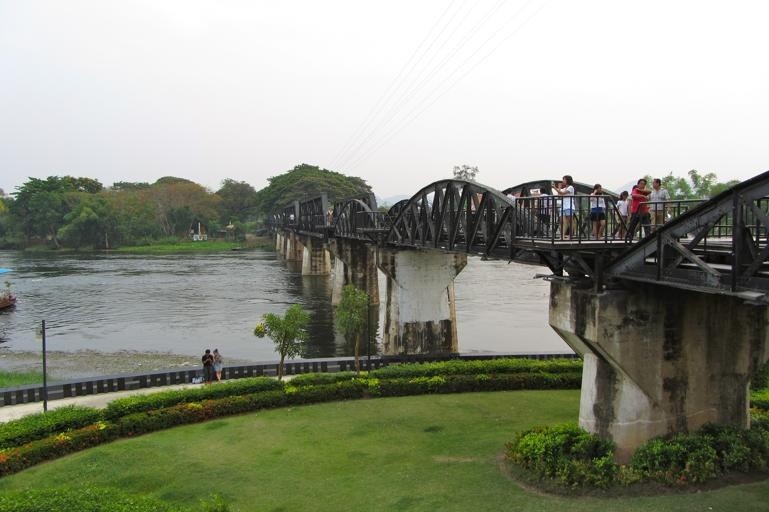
[590, 184, 607, 239]
[506, 176, 575, 241]
[213, 349, 224, 382]
[472, 194, 484, 214]
[202, 349, 213, 384]
[625, 179, 672, 242]
[290, 213, 294, 224]
[327, 208, 334, 225]
[616, 191, 630, 237]
